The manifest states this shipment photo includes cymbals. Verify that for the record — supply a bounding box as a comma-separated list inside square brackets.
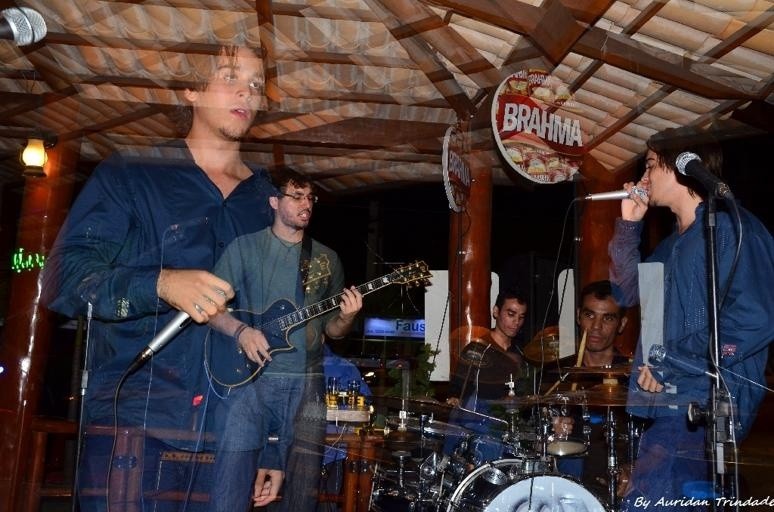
[527, 386, 700, 406]
[481, 396, 539, 406]
[564, 365, 658, 376]
[378, 394, 509, 426]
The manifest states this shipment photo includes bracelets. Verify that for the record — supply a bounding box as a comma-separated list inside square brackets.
[232, 324, 247, 356]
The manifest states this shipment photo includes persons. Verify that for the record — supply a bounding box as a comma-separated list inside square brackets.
[39, 29, 306, 512]
[529, 280, 653, 512]
[309, 332, 374, 512]
[453, 291, 538, 424]
[212, 164, 365, 512]
[608, 129, 774, 512]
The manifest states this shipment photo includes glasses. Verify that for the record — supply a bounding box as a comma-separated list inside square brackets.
[278, 192, 318, 204]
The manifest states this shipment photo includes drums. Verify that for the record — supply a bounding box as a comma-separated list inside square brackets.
[378, 417, 472, 498]
[529, 397, 592, 457]
[446, 460, 613, 512]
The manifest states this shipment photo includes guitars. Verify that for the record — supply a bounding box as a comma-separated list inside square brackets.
[204, 260, 434, 388]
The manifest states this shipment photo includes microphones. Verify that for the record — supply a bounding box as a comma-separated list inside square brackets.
[675, 151, 726, 198]
[0, 7, 49, 47]
[647, 343, 706, 377]
[141, 283, 230, 361]
[584, 186, 646, 202]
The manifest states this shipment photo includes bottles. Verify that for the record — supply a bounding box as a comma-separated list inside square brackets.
[324, 376, 364, 409]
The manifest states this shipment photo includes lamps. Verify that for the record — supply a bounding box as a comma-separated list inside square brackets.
[18, 131, 55, 178]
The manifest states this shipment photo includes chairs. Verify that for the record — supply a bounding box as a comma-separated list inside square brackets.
[24, 420, 422, 512]
